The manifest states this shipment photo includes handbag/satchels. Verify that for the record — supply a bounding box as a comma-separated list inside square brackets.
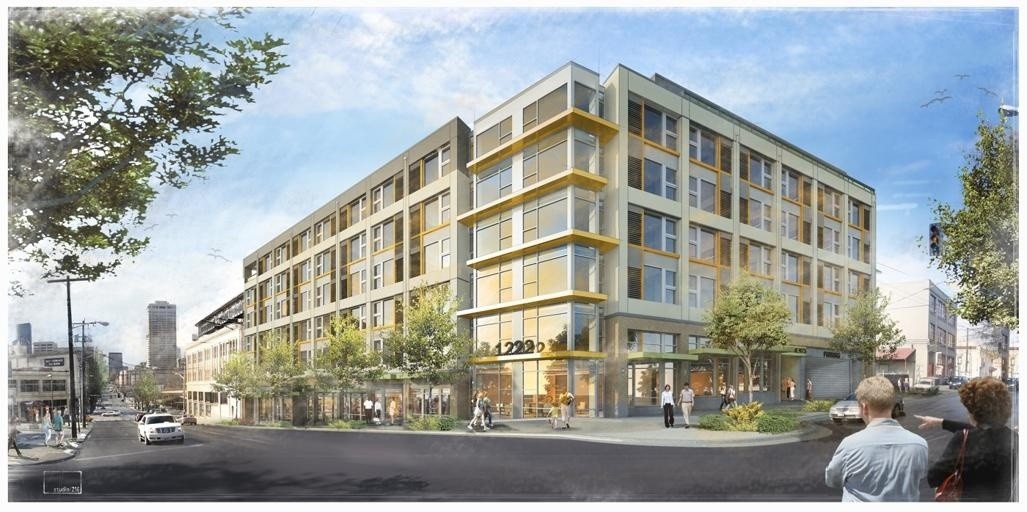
[935, 428, 970, 502]
[561, 392, 571, 406]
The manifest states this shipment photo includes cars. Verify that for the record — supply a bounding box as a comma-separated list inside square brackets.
[1006, 379, 1020, 391]
[137, 414, 182, 446]
[828, 390, 905, 423]
[914, 379, 939, 395]
[102, 411, 120, 416]
[948, 375, 969, 390]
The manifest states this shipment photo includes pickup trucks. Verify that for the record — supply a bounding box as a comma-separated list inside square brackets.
[182, 415, 197, 425]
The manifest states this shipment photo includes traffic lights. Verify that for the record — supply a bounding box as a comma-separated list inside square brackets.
[929, 223, 944, 257]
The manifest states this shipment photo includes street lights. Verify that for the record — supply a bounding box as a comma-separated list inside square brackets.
[998, 105, 1018, 118]
[82, 321, 109, 428]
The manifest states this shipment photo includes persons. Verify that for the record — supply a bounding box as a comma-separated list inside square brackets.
[387, 395, 397, 425]
[717, 380, 728, 412]
[788, 377, 797, 400]
[812, 374, 930, 502]
[660, 383, 675, 428]
[677, 381, 695, 428]
[806, 377, 813, 400]
[546, 401, 560, 429]
[784, 377, 790, 399]
[722, 383, 737, 412]
[466, 392, 490, 430]
[8, 403, 70, 456]
[374, 397, 383, 419]
[557, 386, 575, 429]
[363, 396, 374, 424]
[913, 374, 1018, 502]
[480, 391, 493, 427]
[470, 389, 481, 426]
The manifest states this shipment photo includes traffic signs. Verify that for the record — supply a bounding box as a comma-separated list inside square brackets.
[43, 357, 64, 366]
